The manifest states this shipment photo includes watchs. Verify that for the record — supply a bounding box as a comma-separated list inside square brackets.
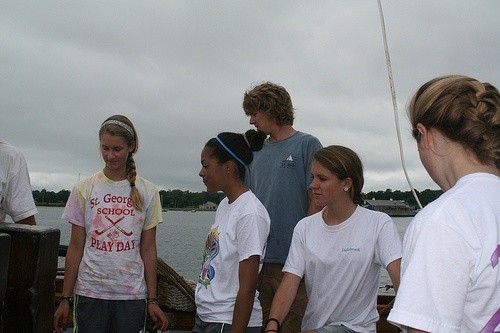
[59, 295, 73, 303]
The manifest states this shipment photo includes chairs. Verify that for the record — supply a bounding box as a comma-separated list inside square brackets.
[157, 257, 196, 312]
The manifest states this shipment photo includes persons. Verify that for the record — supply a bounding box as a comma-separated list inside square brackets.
[0, 140, 38, 226]
[54, 115, 169, 333]
[241, 82, 323, 333]
[193, 130, 267, 333]
[387, 73, 500, 333]
[265, 145, 402, 333]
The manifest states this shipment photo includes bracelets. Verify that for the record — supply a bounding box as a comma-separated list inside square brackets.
[148, 298, 160, 305]
[266, 314, 280, 331]
